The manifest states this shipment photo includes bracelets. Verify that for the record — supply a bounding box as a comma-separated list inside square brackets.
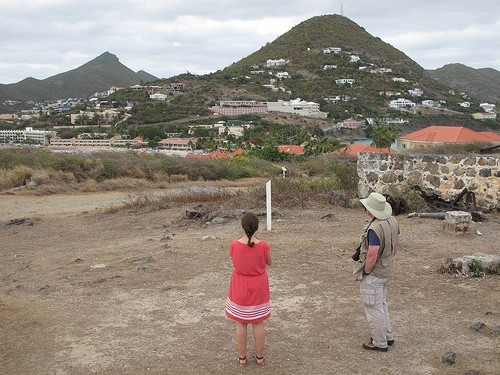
[363, 269, 369, 276]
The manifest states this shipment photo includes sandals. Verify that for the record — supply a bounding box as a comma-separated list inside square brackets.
[235, 355, 247, 367]
[255, 354, 266, 365]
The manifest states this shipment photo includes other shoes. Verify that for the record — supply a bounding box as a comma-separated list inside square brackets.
[362, 334, 395, 352]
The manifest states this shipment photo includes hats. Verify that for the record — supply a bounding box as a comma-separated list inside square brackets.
[359, 192, 392, 220]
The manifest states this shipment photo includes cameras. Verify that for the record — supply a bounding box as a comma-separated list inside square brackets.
[352, 244, 361, 262]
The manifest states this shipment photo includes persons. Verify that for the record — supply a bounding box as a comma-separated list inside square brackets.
[353, 193, 400, 352]
[226, 213, 272, 367]
